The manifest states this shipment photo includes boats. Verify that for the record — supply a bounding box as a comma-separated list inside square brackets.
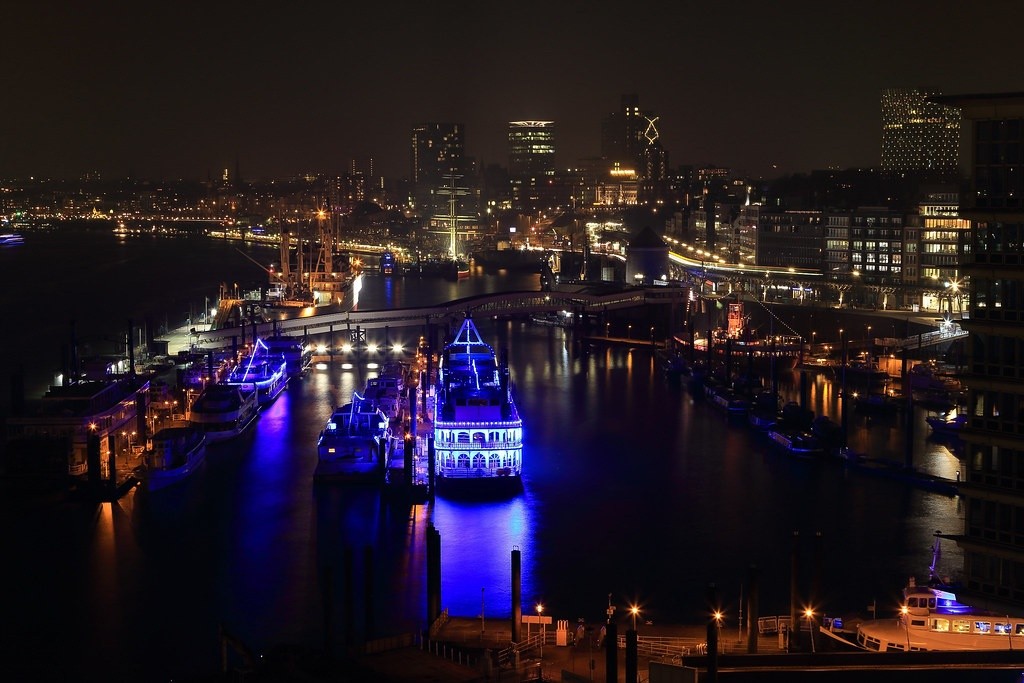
[133, 426, 211, 497]
[214, 325, 421, 484]
[430, 307, 524, 489]
[851, 572, 1024, 655]
[660, 350, 829, 462]
[185, 378, 263, 448]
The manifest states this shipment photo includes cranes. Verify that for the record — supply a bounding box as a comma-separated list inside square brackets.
[235, 206, 334, 297]
